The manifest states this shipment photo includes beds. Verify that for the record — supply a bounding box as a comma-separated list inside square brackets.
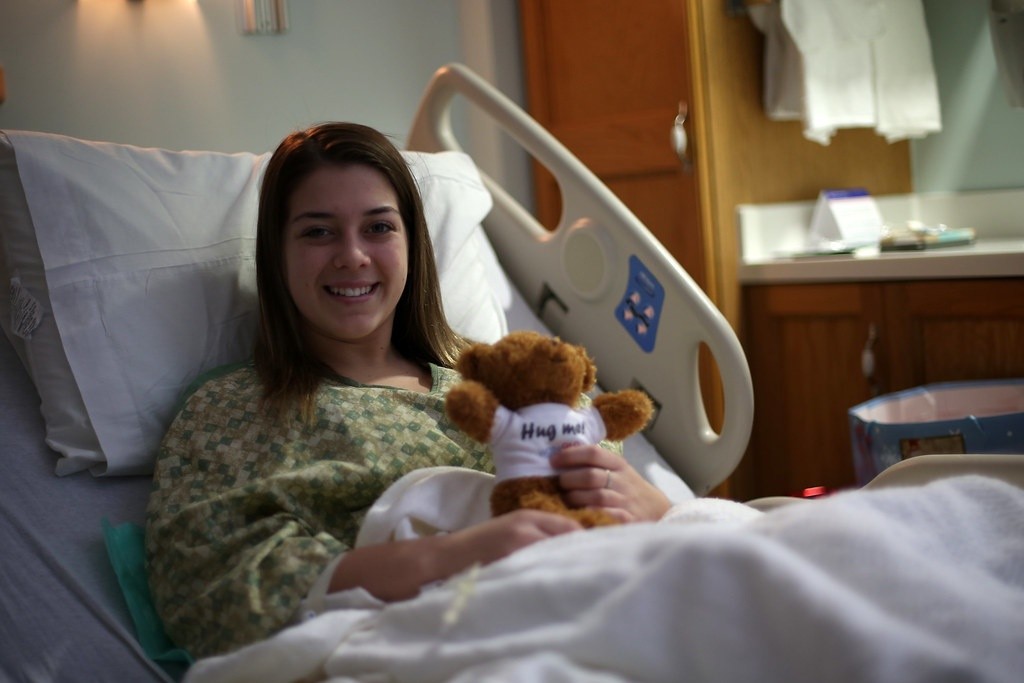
[0, 66, 1023, 683]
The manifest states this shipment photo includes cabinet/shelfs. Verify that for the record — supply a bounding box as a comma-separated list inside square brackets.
[516, 0, 914, 504]
[738, 281, 1024, 502]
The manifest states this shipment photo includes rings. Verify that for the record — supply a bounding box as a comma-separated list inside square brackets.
[605, 470, 611, 488]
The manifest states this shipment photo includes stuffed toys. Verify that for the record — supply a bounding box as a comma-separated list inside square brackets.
[447, 333, 654, 528]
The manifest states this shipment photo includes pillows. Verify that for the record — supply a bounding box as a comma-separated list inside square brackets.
[2, 131, 511, 477]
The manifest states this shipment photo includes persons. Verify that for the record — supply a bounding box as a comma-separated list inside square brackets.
[147, 122, 673, 664]
